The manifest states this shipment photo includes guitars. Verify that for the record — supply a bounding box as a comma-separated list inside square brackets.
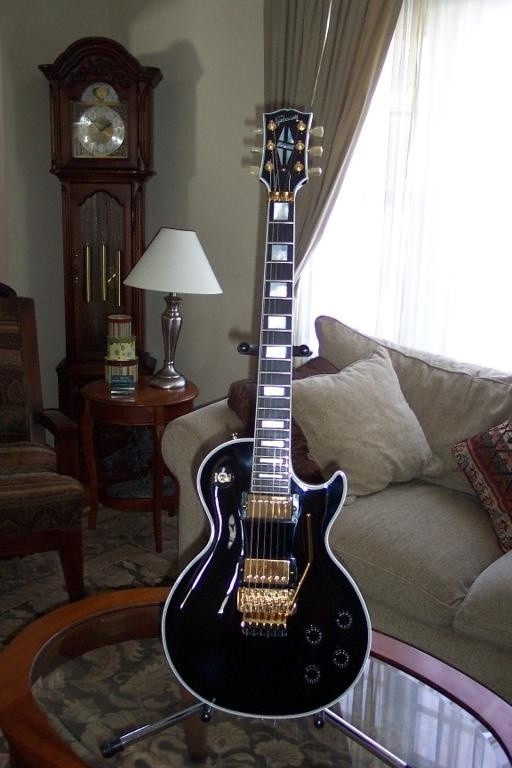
[160, 108, 372, 721]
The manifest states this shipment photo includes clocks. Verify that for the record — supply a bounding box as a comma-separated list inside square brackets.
[38, 35, 163, 184]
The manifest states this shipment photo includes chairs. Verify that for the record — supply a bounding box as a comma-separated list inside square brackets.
[1, 279, 87, 604]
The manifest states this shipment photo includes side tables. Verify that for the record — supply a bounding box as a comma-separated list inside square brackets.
[80, 375, 199, 553]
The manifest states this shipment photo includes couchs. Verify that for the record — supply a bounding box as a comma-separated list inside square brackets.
[162, 315, 512, 707]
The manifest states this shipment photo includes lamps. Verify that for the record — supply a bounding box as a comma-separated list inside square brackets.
[122, 227, 224, 390]
